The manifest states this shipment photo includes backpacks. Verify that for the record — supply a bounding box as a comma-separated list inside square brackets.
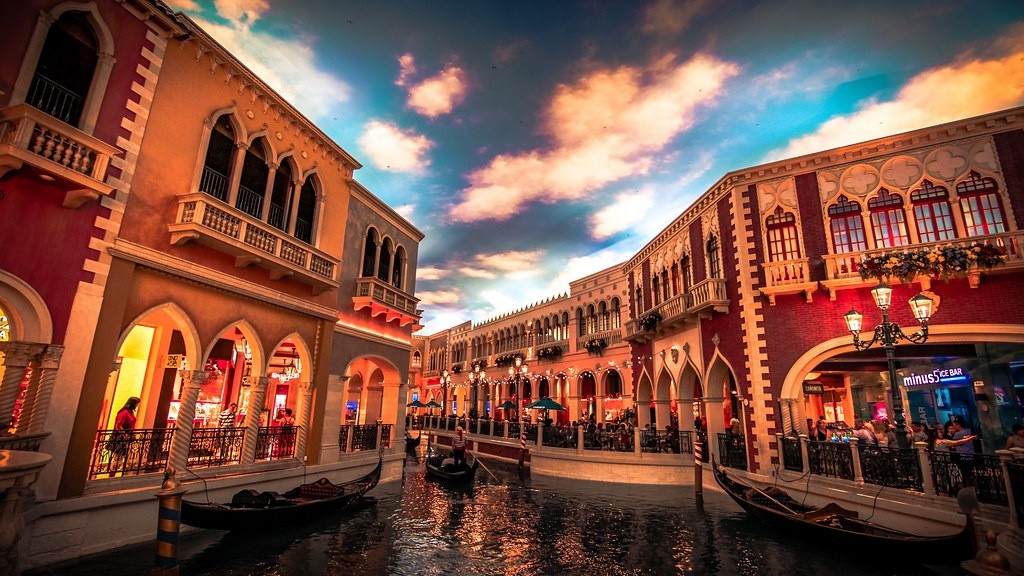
[282, 417, 292, 434]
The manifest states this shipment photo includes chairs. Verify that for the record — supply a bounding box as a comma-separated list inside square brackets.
[641, 436, 679, 454]
[584, 433, 636, 452]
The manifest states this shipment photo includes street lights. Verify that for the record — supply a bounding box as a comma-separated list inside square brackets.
[469, 363, 486, 422]
[439, 368, 450, 417]
[843, 281, 934, 472]
[508, 353, 528, 433]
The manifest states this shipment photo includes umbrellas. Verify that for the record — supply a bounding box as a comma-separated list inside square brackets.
[497, 401, 516, 422]
[524, 397, 567, 421]
[424, 401, 441, 415]
[407, 400, 426, 414]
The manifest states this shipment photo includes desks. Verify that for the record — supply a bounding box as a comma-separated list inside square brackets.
[601, 433, 618, 451]
[647, 435, 665, 451]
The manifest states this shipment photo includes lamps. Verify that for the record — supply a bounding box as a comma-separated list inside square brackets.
[241, 338, 253, 361]
[671, 349, 679, 363]
[272, 348, 302, 383]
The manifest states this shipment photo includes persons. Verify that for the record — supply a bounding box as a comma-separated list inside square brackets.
[904, 414, 979, 493]
[807, 414, 902, 487]
[105, 397, 141, 477]
[273, 408, 295, 456]
[584, 409, 636, 451]
[725, 417, 740, 468]
[217, 403, 238, 462]
[451, 425, 467, 473]
[694, 417, 709, 463]
[1004, 423, 1024, 450]
[640, 413, 680, 453]
[509, 416, 585, 448]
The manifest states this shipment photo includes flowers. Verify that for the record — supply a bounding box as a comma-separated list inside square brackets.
[636, 312, 660, 332]
[452, 365, 462, 373]
[856, 242, 1003, 282]
[494, 354, 524, 368]
[584, 338, 609, 357]
[536, 346, 563, 363]
[471, 359, 488, 373]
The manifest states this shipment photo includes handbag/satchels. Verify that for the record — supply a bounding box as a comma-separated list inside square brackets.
[106, 430, 126, 456]
[214, 429, 220, 438]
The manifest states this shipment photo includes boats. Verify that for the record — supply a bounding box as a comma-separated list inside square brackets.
[407, 429, 421, 450]
[711, 452, 979, 564]
[162, 456, 383, 531]
[425, 453, 479, 483]
